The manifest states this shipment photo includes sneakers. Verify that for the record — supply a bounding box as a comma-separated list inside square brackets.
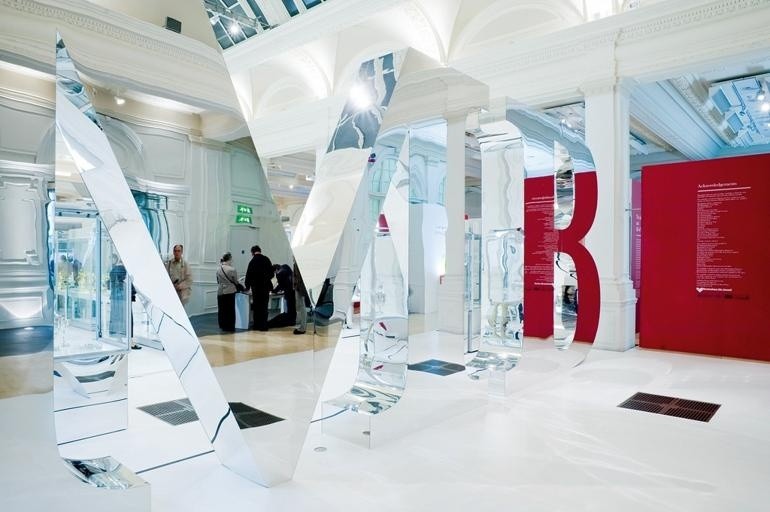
[132, 345, 141, 349]
[294, 329, 306, 334]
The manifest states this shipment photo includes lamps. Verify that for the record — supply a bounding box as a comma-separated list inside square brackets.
[209, 16, 220, 25]
[114, 95, 125, 106]
[756, 90, 765, 101]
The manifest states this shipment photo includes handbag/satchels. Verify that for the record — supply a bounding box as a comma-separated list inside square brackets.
[236, 282, 244, 291]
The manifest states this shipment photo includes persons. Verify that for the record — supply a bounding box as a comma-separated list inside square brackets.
[245, 245, 274, 331]
[293, 259, 309, 335]
[272, 264, 296, 325]
[109, 260, 142, 350]
[216, 253, 238, 333]
[164, 245, 192, 306]
[315, 278, 334, 317]
[50, 254, 82, 286]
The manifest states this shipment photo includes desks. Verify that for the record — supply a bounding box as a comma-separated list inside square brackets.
[233, 291, 287, 329]
